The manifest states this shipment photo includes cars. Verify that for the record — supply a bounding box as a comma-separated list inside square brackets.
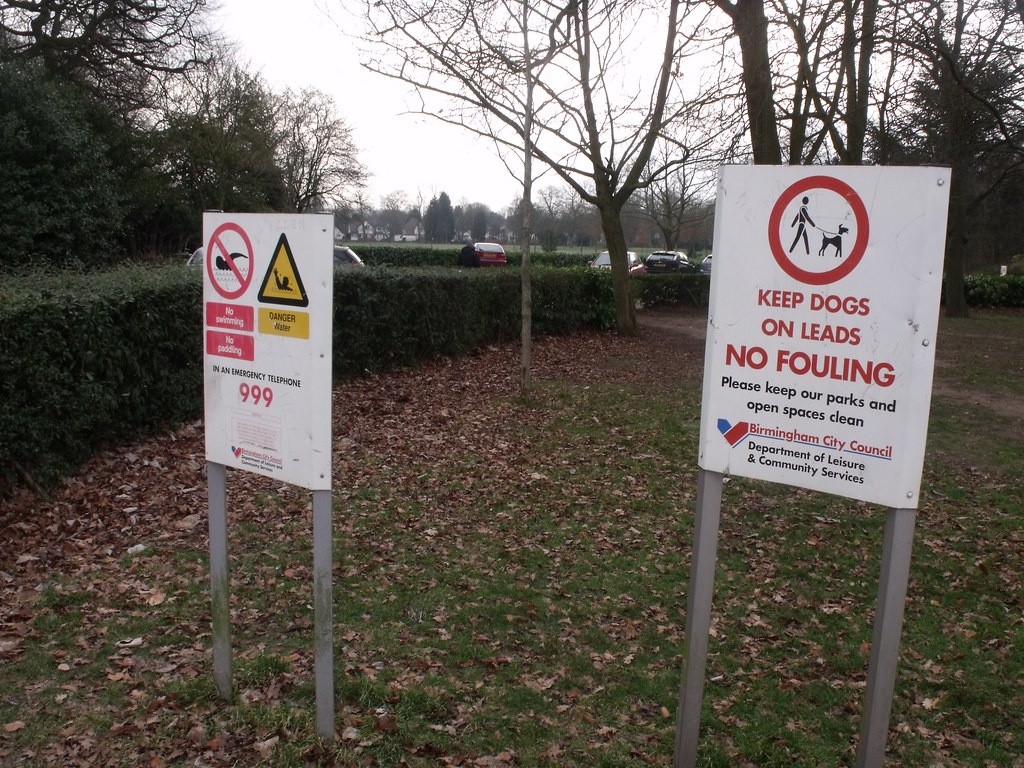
[473, 242, 508, 266]
[185, 245, 365, 266]
[700, 254, 712, 272]
[587, 250, 647, 276]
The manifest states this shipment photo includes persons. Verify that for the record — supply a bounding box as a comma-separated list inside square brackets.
[457, 240, 481, 269]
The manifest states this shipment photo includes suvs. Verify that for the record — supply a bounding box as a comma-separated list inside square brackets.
[644, 251, 695, 275]
